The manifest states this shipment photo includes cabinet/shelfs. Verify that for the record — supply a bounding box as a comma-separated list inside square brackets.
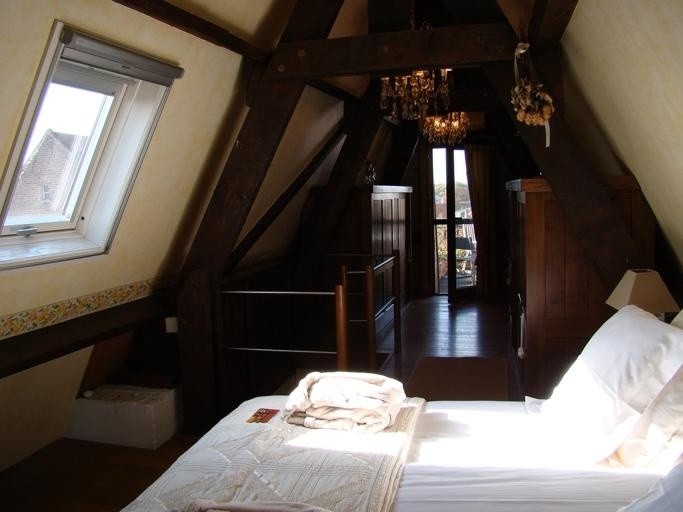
[357, 184, 413, 335]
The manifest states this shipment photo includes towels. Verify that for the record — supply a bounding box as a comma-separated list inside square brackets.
[281, 372, 407, 432]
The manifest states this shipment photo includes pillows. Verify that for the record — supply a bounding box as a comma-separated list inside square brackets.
[522, 306, 683, 474]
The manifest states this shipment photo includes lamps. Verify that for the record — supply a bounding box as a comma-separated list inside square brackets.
[604, 269, 679, 323]
[379, 0, 486, 148]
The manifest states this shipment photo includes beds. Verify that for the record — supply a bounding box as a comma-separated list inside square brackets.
[116, 304, 683, 512]
